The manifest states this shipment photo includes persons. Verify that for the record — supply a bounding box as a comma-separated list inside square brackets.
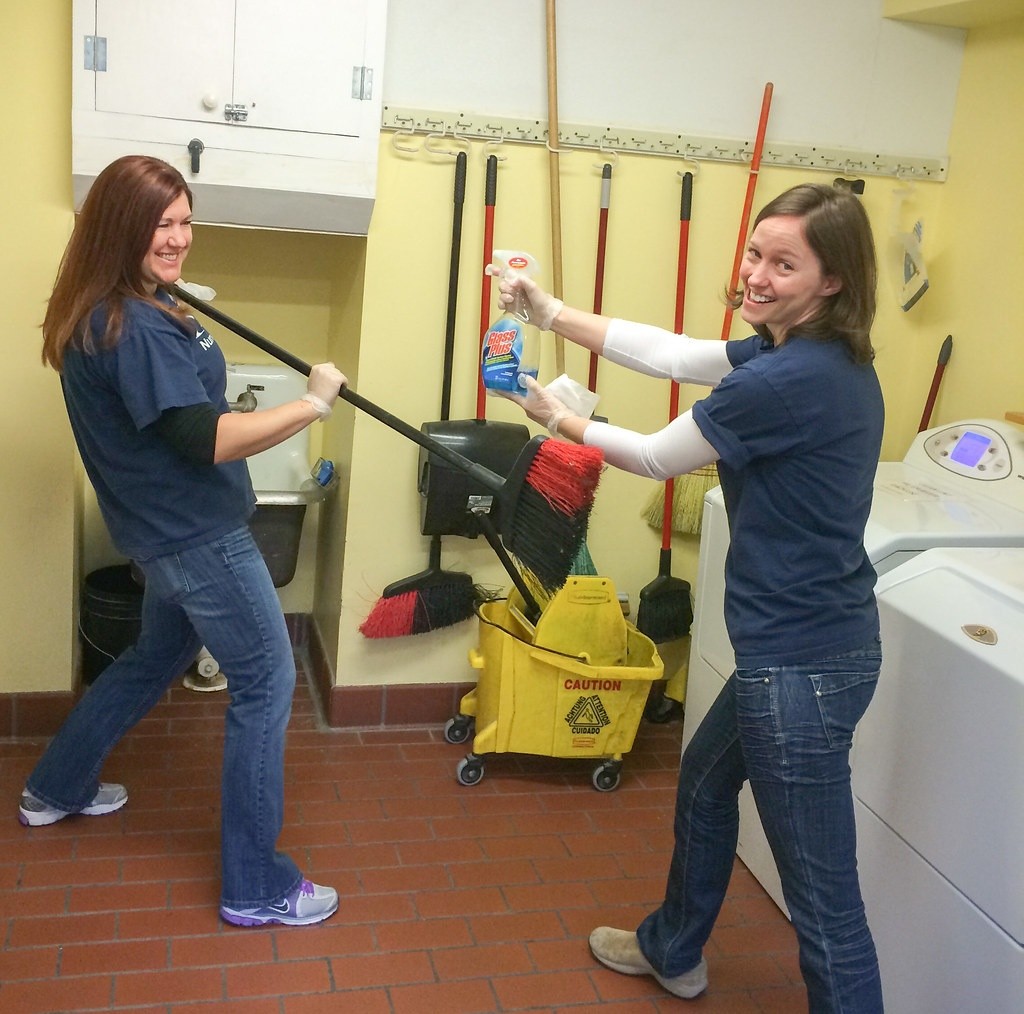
[491, 184, 884, 1014]
[19, 154, 348, 926]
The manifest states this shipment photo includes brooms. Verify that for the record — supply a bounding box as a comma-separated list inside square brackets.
[360, 0, 953, 641]
[167, 280, 601, 591]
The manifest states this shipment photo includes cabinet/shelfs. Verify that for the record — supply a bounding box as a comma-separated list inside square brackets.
[69, 0, 389, 240]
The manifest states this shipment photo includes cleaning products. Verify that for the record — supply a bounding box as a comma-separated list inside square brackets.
[482, 250, 542, 400]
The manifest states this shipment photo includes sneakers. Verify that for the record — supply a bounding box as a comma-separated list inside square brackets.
[18, 779, 128, 825]
[589, 926, 707, 998]
[220, 876, 339, 925]
